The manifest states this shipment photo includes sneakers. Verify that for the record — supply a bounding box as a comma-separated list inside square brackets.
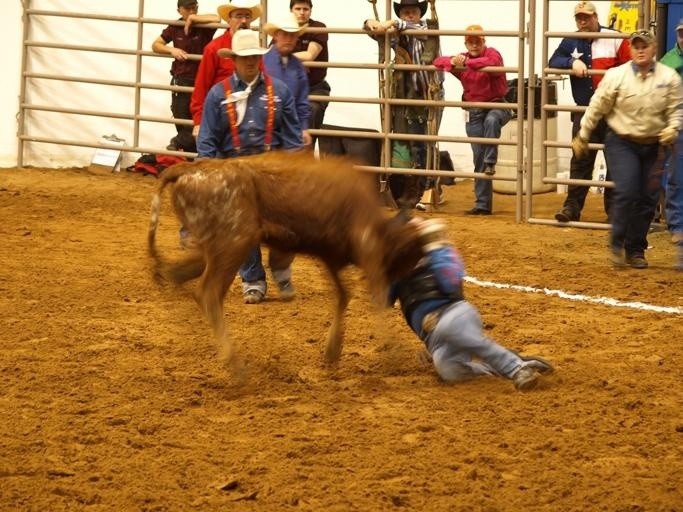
[416, 189, 446, 210]
[608, 248, 626, 266]
[243, 290, 263, 303]
[625, 253, 648, 268]
[278, 280, 295, 299]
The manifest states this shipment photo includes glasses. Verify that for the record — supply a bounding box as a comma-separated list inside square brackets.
[631, 32, 651, 37]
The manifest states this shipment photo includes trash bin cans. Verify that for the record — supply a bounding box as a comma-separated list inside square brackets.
[492, 78, 558, 194]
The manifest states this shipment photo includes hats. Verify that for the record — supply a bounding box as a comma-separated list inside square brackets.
[178, 0, 198, 8]
[631, 31, 654, 43]
[574, 1, 597, 16]
[216, 29, 273, 58]
[217, 0, 262, 24]
[394, 0, 427, 18]
[465, 25, 484, 41]
[262, 13, 309, 36]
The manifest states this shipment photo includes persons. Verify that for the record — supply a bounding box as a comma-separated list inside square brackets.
[571, 30, 683, 268]
[433, 24, 512, 216]
[658, 19, 683, 245]
[549, 2, 632, 223]
[151, 0, 332, 162]
[378, 214, 554, 392]
[195, 29, 299, 302]
[365, 0, 442, 210]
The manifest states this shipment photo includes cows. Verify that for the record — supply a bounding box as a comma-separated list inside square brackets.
[146, 147, 447, 364]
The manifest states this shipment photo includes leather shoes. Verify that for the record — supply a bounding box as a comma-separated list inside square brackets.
[485, 164, 495, 173]
[555, 205, 580, 222]
[507, 348, 554, 371]
[464, 207, 492, 215]
[513, 366, 541, 388]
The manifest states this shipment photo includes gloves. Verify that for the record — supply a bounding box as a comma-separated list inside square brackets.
[573, 133, 589, 160]
[658, 127, 679, 146]
